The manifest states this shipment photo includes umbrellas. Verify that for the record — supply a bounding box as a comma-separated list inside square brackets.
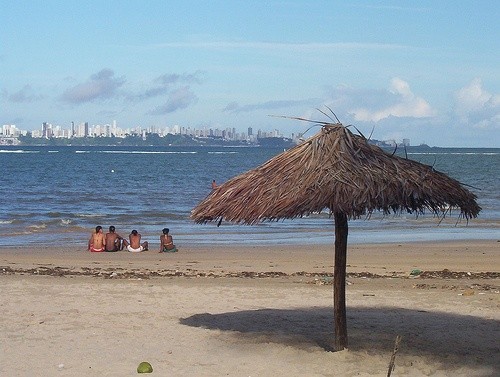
[191, 105, 483, 352]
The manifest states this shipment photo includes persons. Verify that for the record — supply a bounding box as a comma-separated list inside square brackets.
[88, 225, 106, 252]
[105, 226, 123, 252]
[123, 230, 148, 252]
[158, 228, 178, 253]
[212, 180, 216, 188]
